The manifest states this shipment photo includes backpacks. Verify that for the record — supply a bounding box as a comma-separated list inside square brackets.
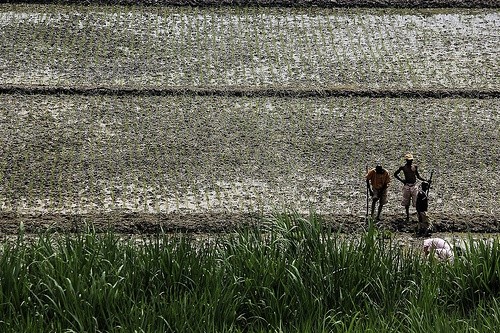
[416, 190, 427, 212]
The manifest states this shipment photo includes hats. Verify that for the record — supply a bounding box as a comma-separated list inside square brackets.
[375, 166, 383, 170]
[404, 154, 414, 161]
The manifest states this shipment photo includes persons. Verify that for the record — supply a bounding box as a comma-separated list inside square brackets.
[394, 154, 432, 222]
[415, 182, 434, 237]
[365, 163, 392, 221]
